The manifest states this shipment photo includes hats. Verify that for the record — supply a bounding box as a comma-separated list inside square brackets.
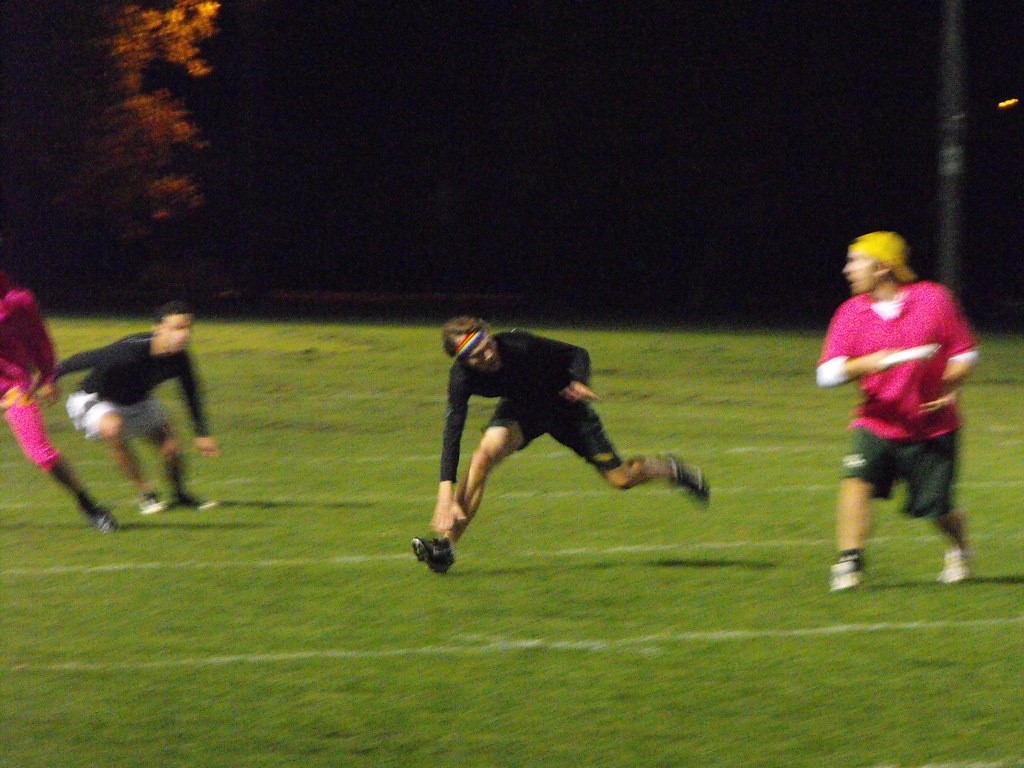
[848, 228, 920, 283]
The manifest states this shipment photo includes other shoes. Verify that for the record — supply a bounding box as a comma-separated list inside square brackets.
[663, 452, 710, 506]
[828, 549, 866, 593]
[87, 504, 121, 535]
[411, 534, 455, 574]
[139, 493, 171, 516]
[177, 492, 219, 512]
[941, 547, 975, 583]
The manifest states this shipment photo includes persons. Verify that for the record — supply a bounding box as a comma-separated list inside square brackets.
[42, 301, 220, 515]
[815, 233, 980, 594]
[411, 315, 711, 576]
[0, 270, 119, 534]
